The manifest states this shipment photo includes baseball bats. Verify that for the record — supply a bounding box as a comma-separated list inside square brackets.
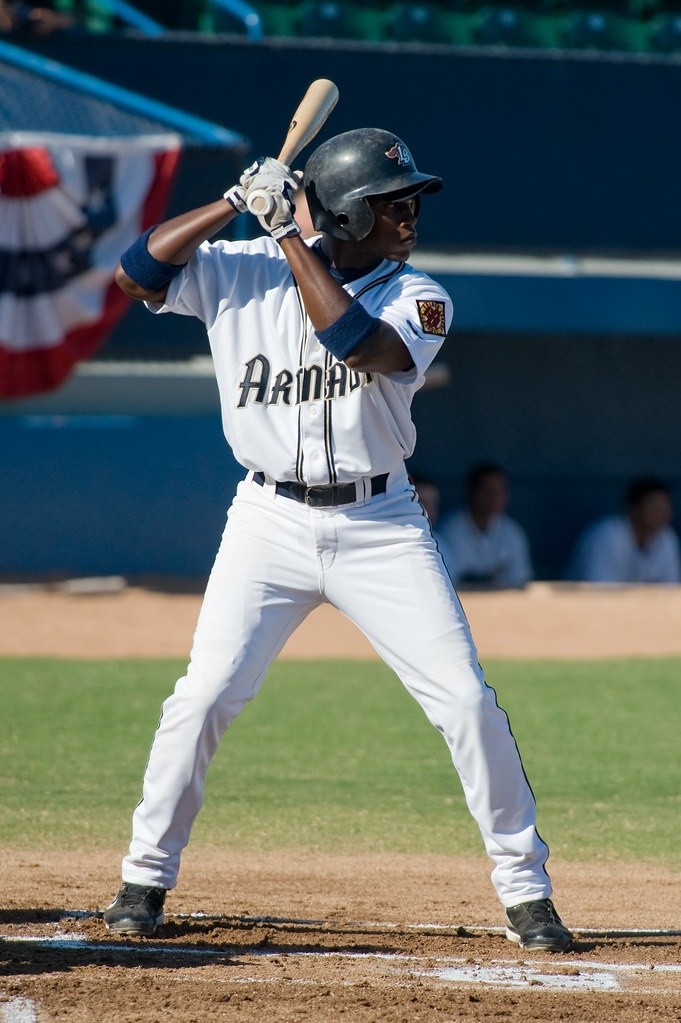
[247, 79, 340, 218]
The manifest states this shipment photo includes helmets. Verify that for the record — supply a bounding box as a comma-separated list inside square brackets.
[303, 128, 442, 241]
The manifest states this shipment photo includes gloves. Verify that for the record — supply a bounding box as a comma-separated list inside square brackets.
[223, 156, 304, 243]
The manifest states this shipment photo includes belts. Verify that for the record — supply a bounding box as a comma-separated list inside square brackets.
[252, 472, 390, 510]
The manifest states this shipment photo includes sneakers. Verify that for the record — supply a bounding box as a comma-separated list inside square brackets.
[505, 897, 574, 952]
[103, 883, 167, 936]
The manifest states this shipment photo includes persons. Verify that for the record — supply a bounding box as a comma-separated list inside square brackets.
[567, 475, 680, 583]
[101, 123, 574, 952]
[439, 462, 534, 588]
[404, 468, 458, 590]
